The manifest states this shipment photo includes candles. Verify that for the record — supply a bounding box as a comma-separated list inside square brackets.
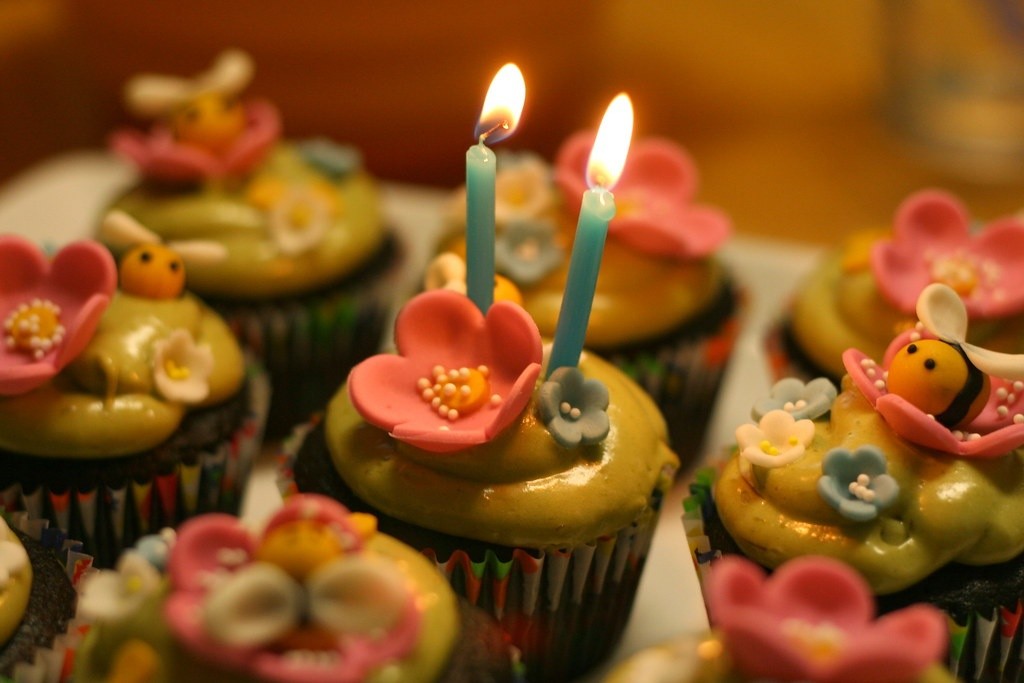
[541, 95, 636, 365]
[466, 60, 527, 311]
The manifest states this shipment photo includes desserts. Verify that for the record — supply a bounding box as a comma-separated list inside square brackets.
[0, 48, 1024, 683]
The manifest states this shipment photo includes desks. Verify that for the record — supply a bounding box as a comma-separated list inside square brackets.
[0, 152, 821, 683]
